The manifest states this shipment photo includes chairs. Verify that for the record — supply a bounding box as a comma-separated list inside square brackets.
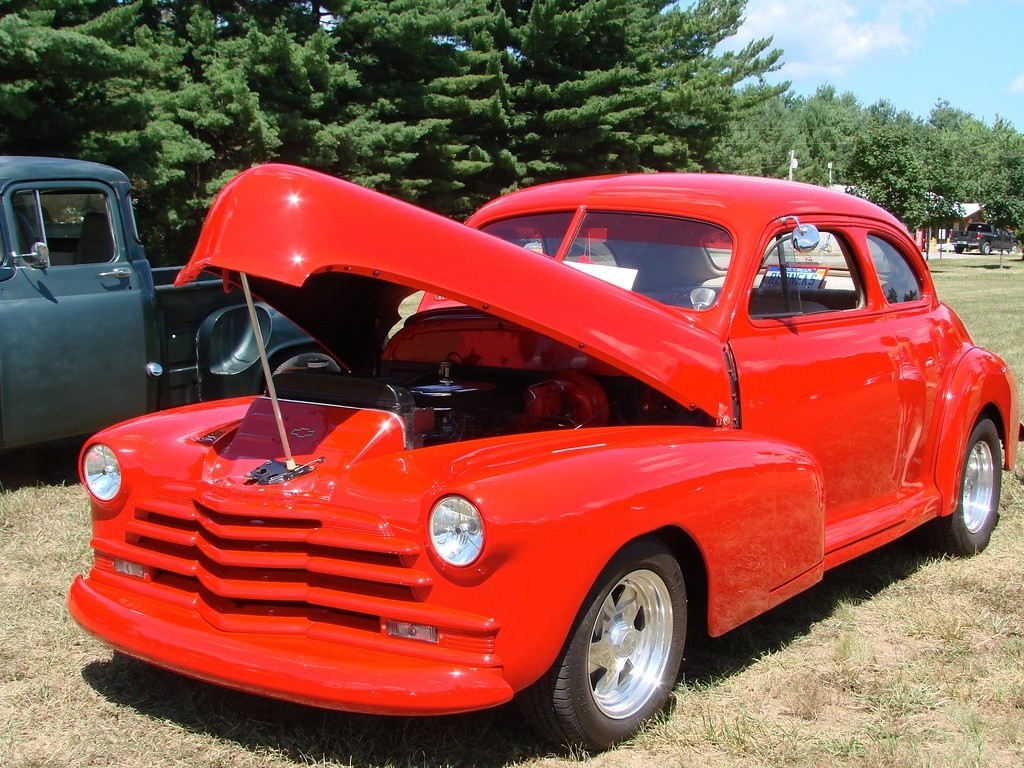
[74, 212, 113, 264]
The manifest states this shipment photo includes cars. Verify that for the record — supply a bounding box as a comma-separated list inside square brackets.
[67, 162, 1024, 748]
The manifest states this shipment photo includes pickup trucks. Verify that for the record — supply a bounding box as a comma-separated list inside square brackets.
[948, 222, 1013, 255]
[0, 156, 351, 455]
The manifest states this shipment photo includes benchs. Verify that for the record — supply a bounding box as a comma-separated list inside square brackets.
[661, 287, 856, 315]
